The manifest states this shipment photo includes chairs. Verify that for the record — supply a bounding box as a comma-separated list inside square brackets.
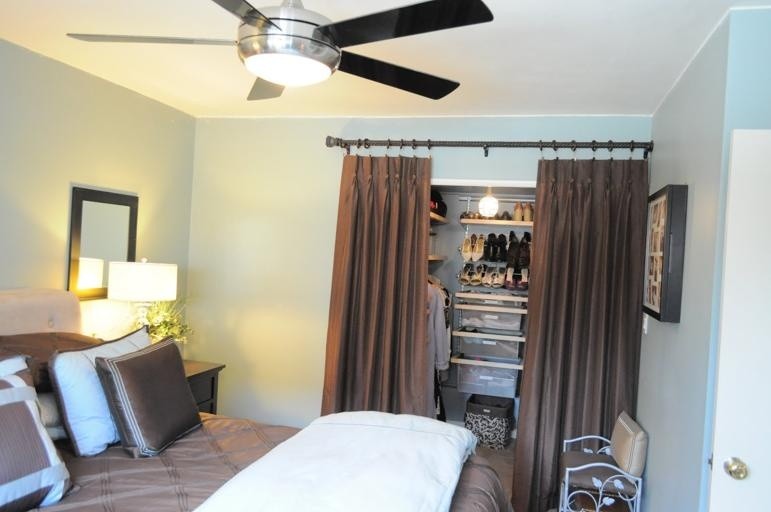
[558, 412, 648, 511]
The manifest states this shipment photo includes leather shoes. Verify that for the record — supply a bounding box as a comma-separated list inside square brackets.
[507, 231, 520, 266]
[489, 213, 500, 220]
[472, 233, 485, 262]
[502, 211, 511, 221]
[518, 232, 531, 267]
[467, 211, 487, 220]
[523, 202, 534, 222]
[513, 201, 523, 221]
[486, 233, 497, 261]
[462, 236, 472, 262]
[497, 234, 506, 262]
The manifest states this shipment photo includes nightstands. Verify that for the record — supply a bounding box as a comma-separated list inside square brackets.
[183, 356, 227, 414]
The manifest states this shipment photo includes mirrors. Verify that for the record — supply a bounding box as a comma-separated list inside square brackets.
[67, 185, 140, 301]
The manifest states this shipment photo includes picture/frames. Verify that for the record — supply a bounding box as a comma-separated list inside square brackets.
[643, 184, 689, 322]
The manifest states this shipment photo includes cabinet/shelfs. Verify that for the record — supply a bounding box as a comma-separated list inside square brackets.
[450, 199, 535, 370]
[427, 212, 446, 263]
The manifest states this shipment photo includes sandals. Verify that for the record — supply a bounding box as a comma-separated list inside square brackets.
[470, 265, 483, 286]
[460, 262, 472, 285]
[482, 263, 496, 287]
[493, 265, 507, 288]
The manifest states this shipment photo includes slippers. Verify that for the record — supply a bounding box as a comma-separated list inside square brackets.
[518, 268, 530, 290]
[505, 266, 515, 291]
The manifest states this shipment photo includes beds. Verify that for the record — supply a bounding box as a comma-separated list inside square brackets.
[0, 286, 506, 511]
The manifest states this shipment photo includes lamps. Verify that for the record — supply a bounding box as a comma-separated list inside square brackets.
[78, 257, 103, 290]
[238, 37, 340, 90]
[477, 189, 498, 218]
[106, 260, 178, 326]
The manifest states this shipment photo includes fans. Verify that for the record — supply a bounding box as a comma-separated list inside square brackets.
[68, 1, 494, 102]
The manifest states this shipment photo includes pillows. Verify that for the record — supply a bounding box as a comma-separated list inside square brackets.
[0, 368, 73, 511]
[48, 327, 152, 456]
[94, 336, 203, 457]
[0, 354, 26, 380]
